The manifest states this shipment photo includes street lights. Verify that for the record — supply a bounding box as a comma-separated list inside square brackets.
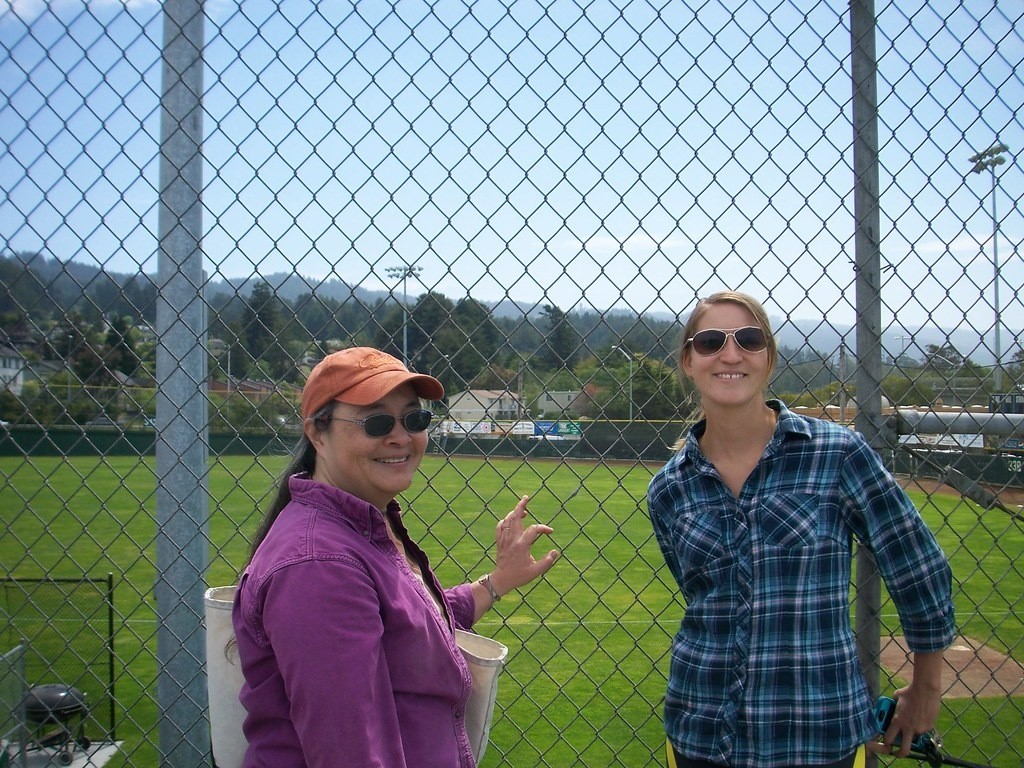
[924, 352, 955, 411]
[67, 335, 75, 409]
[612, 345, 633, 421]
[216, 344, 231, 425]
[384, 266, 424, 367]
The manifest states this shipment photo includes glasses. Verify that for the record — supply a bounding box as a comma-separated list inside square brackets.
[320, 407, 435, 435]
[681, 324, 772, 358]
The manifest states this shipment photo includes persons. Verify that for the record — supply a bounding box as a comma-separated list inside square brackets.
[647, 291, 955, 768]
[233, 346, 558, 768]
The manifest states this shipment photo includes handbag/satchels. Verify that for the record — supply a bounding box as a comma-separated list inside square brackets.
[203, 583, 512, 768]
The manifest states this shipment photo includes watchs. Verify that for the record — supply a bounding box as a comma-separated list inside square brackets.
[479, 574, 501, 602]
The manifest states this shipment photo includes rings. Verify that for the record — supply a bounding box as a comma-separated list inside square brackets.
[503, 527, 510, 530]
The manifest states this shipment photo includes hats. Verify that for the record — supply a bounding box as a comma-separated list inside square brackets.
[301, 344, 446, 416]
